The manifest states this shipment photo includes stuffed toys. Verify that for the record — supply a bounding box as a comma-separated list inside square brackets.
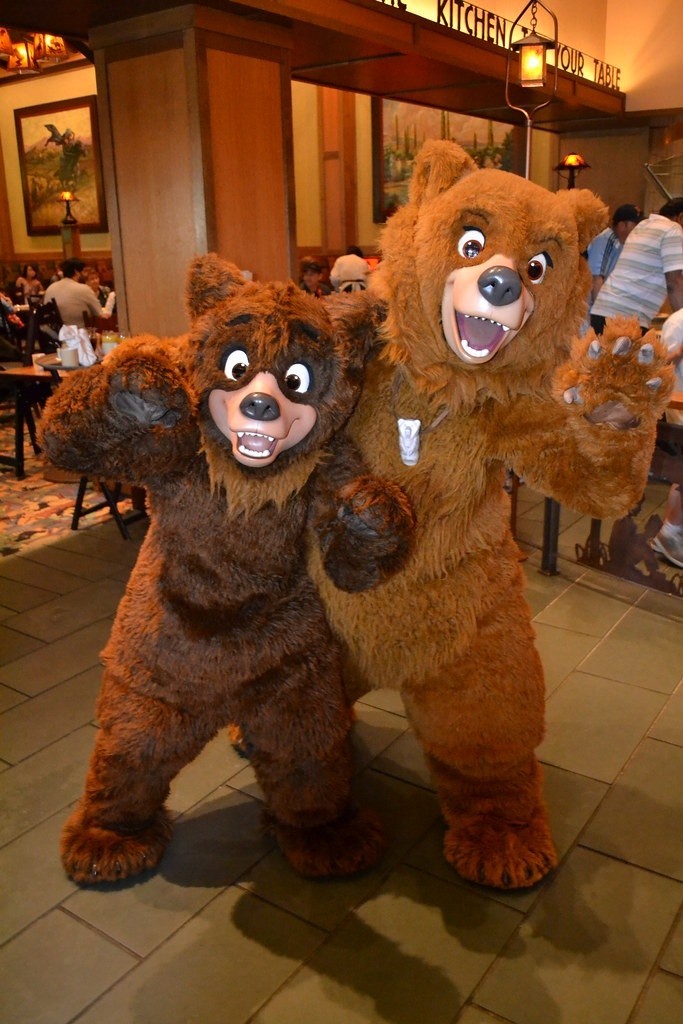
[227, 138, 676, 889]
[36, 254, 414, 884]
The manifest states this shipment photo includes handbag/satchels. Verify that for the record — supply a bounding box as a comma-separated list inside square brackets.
[59, 323, 96, 367]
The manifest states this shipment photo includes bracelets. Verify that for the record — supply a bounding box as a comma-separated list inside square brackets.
[17, 304, 20, 311]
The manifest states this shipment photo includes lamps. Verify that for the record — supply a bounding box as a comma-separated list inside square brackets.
[554, 151, 592, 189]
[56, 192, 80, 224]
[0, 25, 70, 75]
[512, 2, 554, 87]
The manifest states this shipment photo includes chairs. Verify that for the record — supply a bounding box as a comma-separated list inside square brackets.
[0, 281, 118, 481]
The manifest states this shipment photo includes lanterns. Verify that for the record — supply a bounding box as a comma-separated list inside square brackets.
[0, 27, 14, 56]
[33, 33, 68, 63]
[7, 42, 40, 75]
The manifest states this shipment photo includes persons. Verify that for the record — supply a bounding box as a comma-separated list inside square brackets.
[589, 196, 683, 334]
[0, 289, 57, 338]
[587, 203, 647, 313]
[298, 256, 331, 297]
[82, 267, 117, 314]
[16, 262, 43, 304]
[650, 308, 683, 568]
[46, 259, 64, 284]
[329, 246, 370, 293]
[44, 257, 115, 328]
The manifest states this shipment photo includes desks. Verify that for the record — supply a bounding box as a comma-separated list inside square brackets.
[1, 361, 69, 396]
[36, 353, 149, 541]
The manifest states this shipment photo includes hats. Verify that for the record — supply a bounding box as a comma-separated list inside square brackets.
[304, 263, 321, 272]
[611, 205, 644, 221]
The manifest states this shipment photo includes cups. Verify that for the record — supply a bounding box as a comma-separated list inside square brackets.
[60, 348, 79, 368]
[102, 331, 118, 356]
[118, 330, 131, 346]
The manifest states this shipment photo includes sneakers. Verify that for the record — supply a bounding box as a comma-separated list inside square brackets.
[648, 533, 683, 567]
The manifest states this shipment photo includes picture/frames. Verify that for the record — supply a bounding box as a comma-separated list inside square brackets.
[371, 95, 526, 226]
[14, 94, 108, 236]
